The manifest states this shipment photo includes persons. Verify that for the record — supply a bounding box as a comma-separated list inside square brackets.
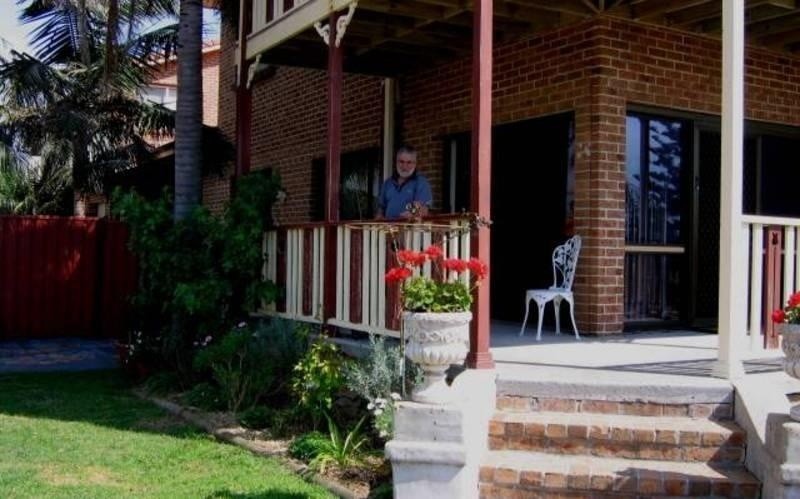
[374, 146, 436, 221]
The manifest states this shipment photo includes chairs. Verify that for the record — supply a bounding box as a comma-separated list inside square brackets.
[520, 234, 582, 341]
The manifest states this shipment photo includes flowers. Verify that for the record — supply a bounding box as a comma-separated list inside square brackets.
[385, 246, 489, 313]
[773, 291, 800, 327]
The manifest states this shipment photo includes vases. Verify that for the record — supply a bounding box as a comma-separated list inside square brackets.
[403, 310, 473, 404]
[112, 339, 151, 380]
[778, 323, 800, 422]
